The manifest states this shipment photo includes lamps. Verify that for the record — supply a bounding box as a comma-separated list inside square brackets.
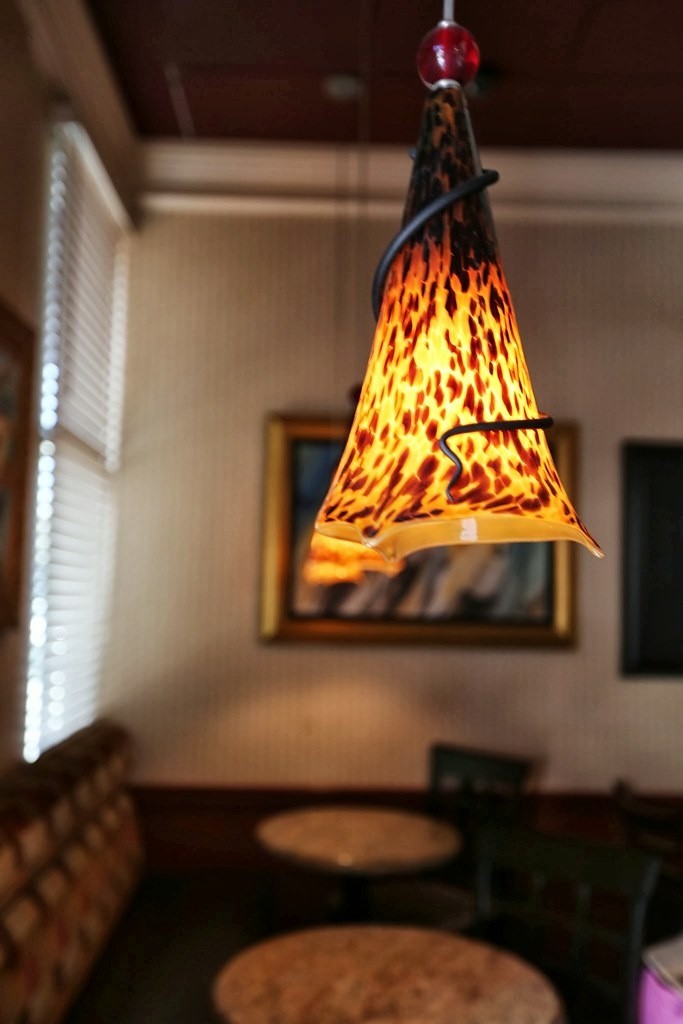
[299, 0, 606, 587]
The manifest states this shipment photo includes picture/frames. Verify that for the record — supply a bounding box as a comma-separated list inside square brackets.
[258, 411, 582, 649]
[620, 436, 683, 681]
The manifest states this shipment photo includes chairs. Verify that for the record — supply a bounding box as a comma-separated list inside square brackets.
[473, 821, 662, 1024]
[615, 783, 683, 943]
[324, 747, 533, 932]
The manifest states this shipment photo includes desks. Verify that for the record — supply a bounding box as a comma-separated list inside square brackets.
[252, 802, 464, 921]
[214, 923, 569, 1024]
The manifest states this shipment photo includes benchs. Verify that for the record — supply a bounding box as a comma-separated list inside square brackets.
[0, 723, 279, 1024]
